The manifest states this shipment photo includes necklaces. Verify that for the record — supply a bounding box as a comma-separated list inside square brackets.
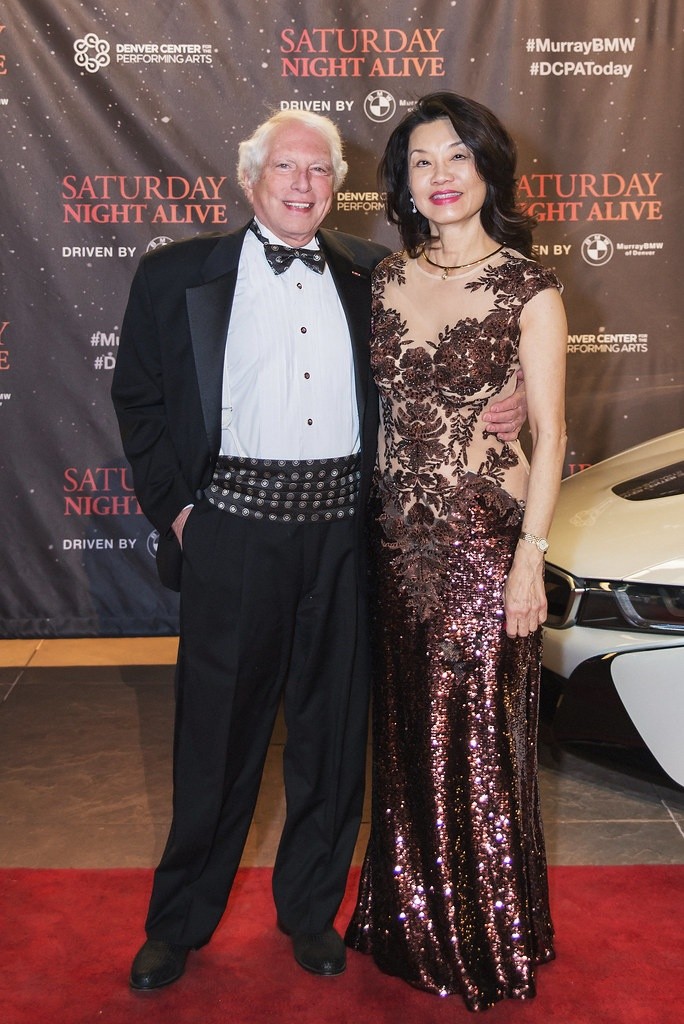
[418, 239, 506, 279]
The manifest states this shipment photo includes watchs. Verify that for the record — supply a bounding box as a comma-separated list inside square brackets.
[516, 531, 549, 553]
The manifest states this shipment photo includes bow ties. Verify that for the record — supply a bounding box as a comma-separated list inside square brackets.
[248, 219, 326, 276]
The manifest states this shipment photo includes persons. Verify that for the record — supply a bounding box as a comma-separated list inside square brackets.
[110, 109, 528, 993]
[343, 92, 567, 1012]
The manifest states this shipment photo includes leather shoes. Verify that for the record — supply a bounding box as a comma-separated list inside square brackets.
[129, 937, 192, 990]
[292, 924, 346, 976]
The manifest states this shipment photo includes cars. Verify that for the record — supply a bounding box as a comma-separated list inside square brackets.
[537, 421, 684, 795]
